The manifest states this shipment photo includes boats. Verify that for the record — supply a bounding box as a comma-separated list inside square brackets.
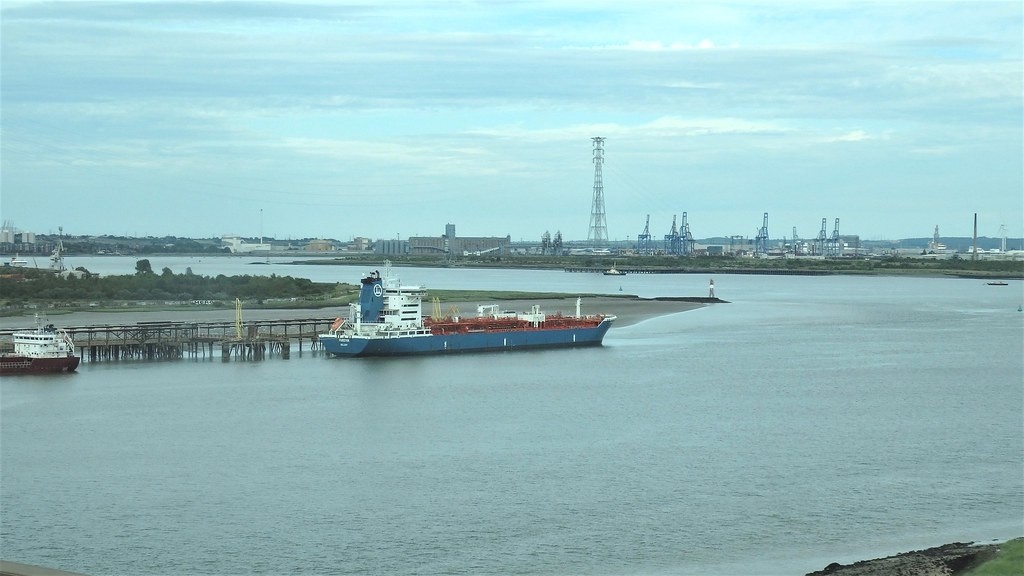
[10, 257, 28, 267]
[318, 258, 618, 357]
[602, 268, 627, 276]
[0, 311, 81, 375]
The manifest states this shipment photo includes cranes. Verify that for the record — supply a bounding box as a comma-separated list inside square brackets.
[637, 212, 843, 257]
